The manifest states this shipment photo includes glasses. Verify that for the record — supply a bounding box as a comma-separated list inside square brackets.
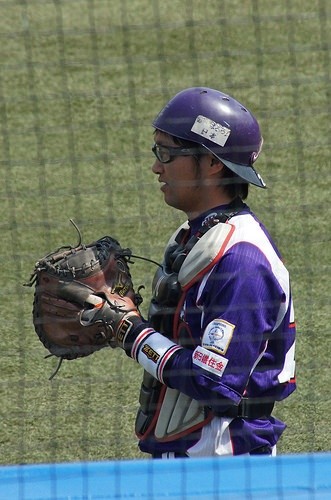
[152, 143, 202, 163]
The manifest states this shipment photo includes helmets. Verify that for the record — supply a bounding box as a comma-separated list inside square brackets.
[151, 87, 268, 189]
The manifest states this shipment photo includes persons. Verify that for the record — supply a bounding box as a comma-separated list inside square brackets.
[58, 87, 297, 458]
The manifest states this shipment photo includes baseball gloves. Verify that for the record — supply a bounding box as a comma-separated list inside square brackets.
[22, 218, 165, 381]
